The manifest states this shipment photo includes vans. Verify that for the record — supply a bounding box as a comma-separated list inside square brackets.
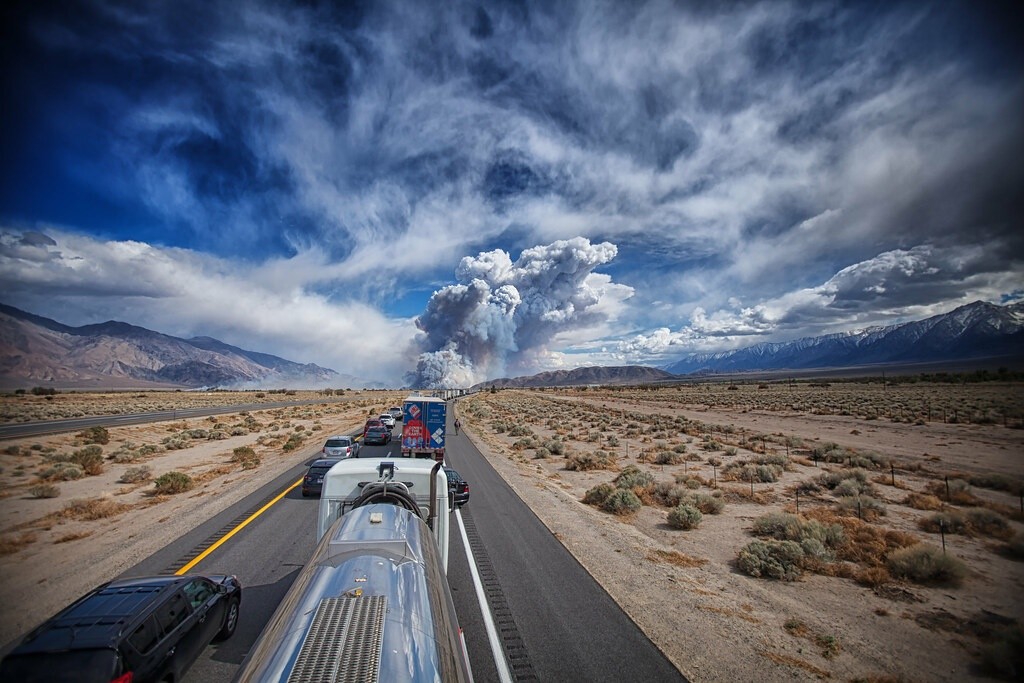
[323, 436, 360, 459]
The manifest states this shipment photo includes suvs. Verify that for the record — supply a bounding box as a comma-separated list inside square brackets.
[388, 407, 402, 419]
[379, 414, 396, 429]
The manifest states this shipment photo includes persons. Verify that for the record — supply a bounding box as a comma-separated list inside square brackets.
[354, 441, 359, 457]
[454, 419, 460, 435]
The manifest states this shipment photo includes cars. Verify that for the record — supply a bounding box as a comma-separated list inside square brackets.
[363, 425, 391, 444]
[444, 468, 470, 507]
[0, 573, 241, 683]
[303, 459, 340, 496]
[362, 419, 383, 431]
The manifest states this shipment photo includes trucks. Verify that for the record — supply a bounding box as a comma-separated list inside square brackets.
[402, 396, 447, 463]
[230, 458, 475, 683]
[437, 388, 468, 401]
